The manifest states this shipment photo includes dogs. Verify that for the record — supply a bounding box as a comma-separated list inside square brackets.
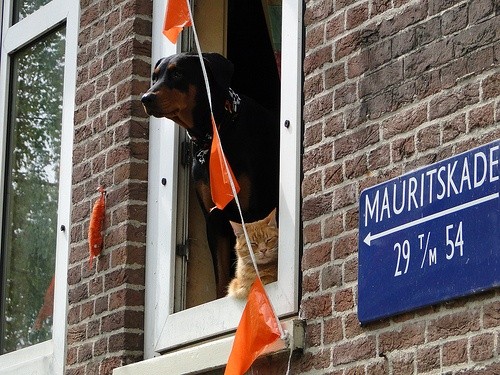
[140, 50, 279, 299]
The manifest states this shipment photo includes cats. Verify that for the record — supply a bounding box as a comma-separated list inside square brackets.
[224, 207, 279, 295]
